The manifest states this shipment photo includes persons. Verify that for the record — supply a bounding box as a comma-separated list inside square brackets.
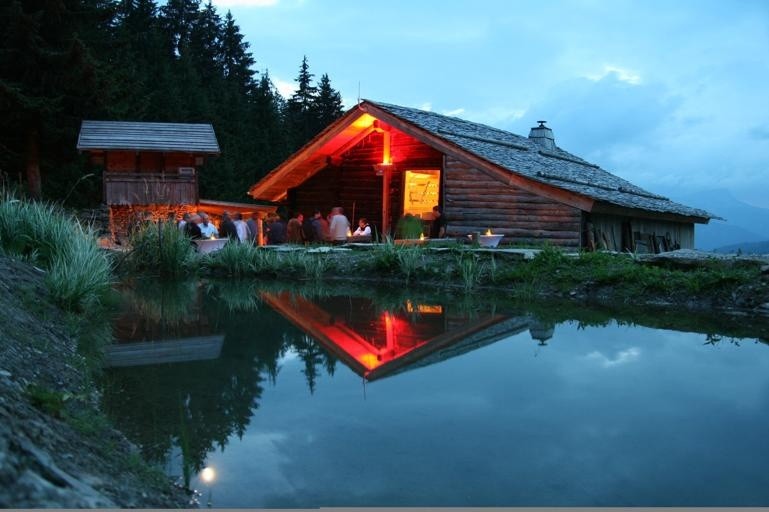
[394, 212, 423, 241]
[431, 205, 447, 237]
[166, 204, 374, 250]
